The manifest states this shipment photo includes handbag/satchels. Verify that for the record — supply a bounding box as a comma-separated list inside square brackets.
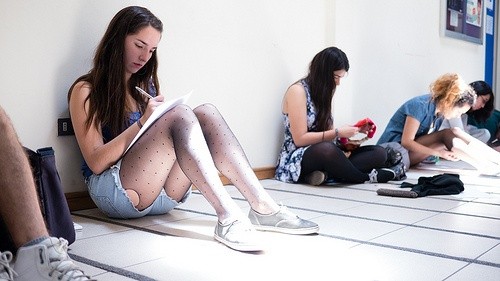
[0, 145, 75, 261]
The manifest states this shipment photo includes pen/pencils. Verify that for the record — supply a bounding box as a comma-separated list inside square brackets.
[135, 86, 153, 98]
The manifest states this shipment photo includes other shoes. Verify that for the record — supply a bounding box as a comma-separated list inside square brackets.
[421, 156, 436, 164]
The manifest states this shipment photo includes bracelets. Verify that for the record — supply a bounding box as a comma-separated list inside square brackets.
[322, 132, 324, 142]
[137, 120, 142, 128]
[335, 128, 338, 137]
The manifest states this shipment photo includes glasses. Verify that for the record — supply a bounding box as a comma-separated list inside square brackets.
[480, 95, 488, 105]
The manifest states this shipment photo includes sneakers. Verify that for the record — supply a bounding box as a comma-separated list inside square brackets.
[248, 202, 320, 234]
[363, 167, 396, 184]
[214, 218, 265, 251]
[309, 171, 327, 186]
[0, 237, 99, 281]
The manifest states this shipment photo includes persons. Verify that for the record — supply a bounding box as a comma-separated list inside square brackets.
[274, 47, 396, 185]
[429, 81, 493, 142]
[67, 5, 319, 252]
[0, 105, 93, 281]
[474, 0, 482, 25]
[376, 73, 477, 170]
